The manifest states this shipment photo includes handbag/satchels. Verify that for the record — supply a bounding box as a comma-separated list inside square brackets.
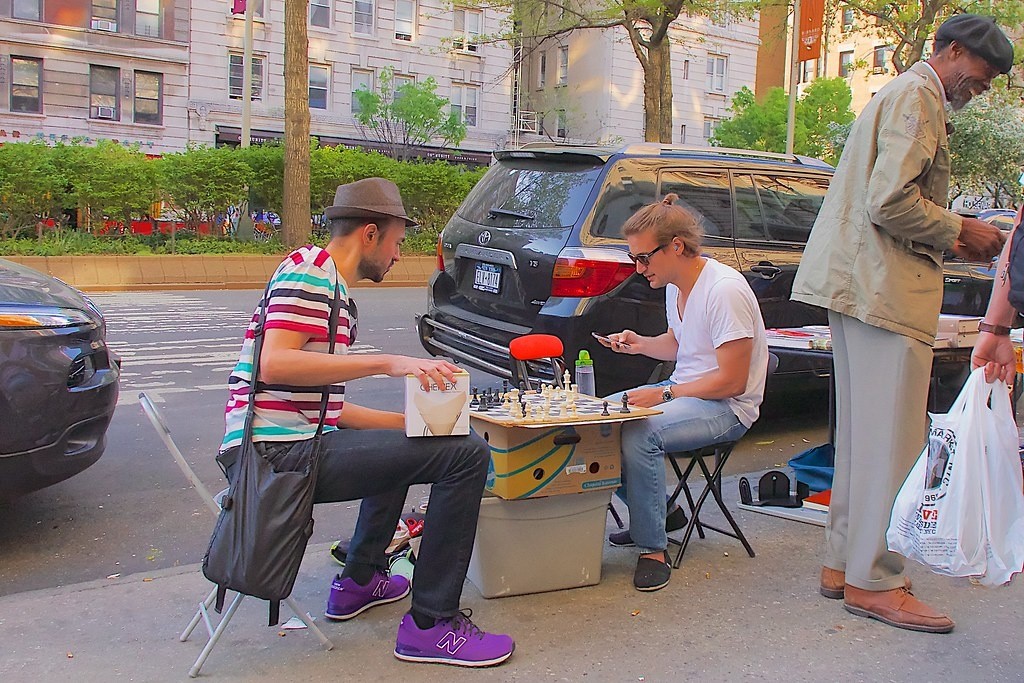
[201, 441, 322, 601]
[886, 366, 1024, 585]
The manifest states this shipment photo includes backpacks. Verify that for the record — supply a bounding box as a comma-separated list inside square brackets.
[1002, 204, 1024, 313]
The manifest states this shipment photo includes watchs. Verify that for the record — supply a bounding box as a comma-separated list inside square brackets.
[662, 385, 673, 402]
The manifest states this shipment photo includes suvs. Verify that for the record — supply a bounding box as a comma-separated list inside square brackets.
[413, 137, 1023, 421]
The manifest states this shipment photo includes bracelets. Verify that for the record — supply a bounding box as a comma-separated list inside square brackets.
[977, 320, 1011, 336]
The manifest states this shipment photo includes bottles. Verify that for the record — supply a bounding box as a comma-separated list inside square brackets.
[575, 349, 596, 398]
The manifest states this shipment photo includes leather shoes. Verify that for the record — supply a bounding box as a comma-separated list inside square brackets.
[820, 566, 912, 599]
[844, 583, 955, 632]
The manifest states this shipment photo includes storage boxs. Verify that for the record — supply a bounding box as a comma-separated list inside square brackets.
[464, 490, 613, 599]
[469, 415, 622, 500]
[403, 370, 470, 438]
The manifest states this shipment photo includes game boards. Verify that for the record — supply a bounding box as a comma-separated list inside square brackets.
[468, 388, 642, 422]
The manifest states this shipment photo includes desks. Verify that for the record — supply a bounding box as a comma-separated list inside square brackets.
[768, 324, 1023, 448]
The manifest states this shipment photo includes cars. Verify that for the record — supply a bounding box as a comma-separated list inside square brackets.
[0, 257, 123, 502]
[970, 209, 1019, 242]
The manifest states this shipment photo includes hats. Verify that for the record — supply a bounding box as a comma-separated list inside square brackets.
[326, 177, 417, 227]
[937, 14, 1014, 73]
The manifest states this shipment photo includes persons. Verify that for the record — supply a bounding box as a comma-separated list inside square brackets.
[219, 179, 515, 669]
[596, 193, 770, 592]
[969, 205, 1024, 385]
[790, 15, 1014, 633]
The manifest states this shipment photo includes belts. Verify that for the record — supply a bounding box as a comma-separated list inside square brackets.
[217, 442, 282, 468]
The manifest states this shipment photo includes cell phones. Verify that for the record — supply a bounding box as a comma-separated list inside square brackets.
[592, 332, 630, 349]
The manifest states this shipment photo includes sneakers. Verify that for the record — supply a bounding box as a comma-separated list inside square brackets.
[634, 550, 672, 590]
[325, 566, 410, 620]
[394, 607, 515, 666]
[609, 505, 688, 546]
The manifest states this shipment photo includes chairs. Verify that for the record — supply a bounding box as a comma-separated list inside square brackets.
[138, 391, 335, 679]
[668, 352, 779, 571]
[509, 333, 624, 534]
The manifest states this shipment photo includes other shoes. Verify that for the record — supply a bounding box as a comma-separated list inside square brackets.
[331, 540, 354, 565]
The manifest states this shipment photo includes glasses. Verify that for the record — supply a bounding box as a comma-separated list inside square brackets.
[628, 235, 677, 264]
